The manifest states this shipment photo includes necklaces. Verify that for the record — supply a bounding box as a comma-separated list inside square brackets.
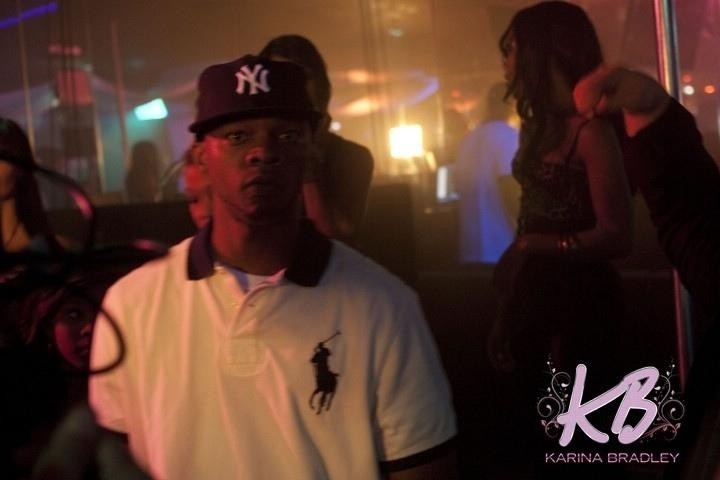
[3, 217, 21, 250]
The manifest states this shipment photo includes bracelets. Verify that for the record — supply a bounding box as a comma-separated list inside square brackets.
[556, 233, 582, 261]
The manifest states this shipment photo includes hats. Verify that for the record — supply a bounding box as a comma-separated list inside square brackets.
[186, 53, 324, 134]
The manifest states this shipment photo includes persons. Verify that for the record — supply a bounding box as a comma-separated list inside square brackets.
[89, 53, 462, 480]
[259, 34, 373, 252]
[124, 135, 167, 206]
[458, 81, 521, 265]
[181, 142, 215, 230]
[571, 65, 719, 479]
[485, 1, 634, 479]
[0, 273, 102, 479]
[1, 115, 82, 253]
